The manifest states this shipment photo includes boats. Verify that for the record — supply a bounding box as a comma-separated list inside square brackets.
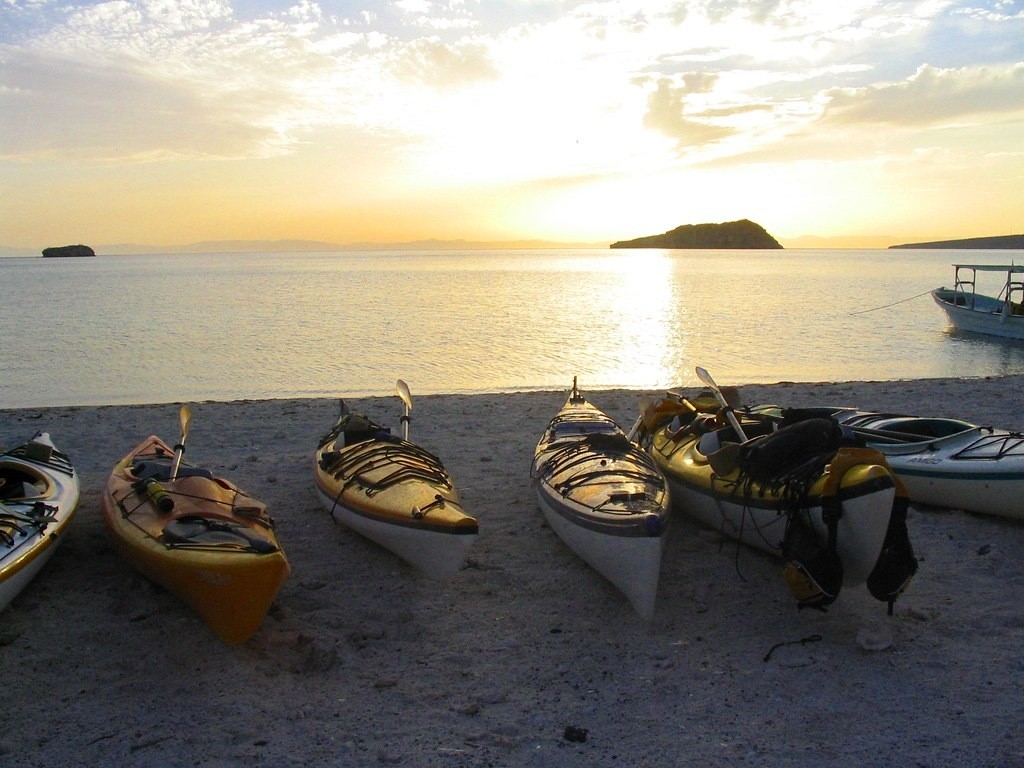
[101, 436, 289, 649]
[314, 395, 481, 582]
[632, 388, 897, 597]
[808, 403, 1024, 524]
[527, 374, 670, 621]
[929, 262, 1024, 341]
[0, 429, 81, 619]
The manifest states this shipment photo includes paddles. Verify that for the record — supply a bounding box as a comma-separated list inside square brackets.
[695, 366, 749, 443]
[666, 391, 701, 417]
[624, 396, 656, 444]
[167, 405, 192, 482]
[396, 379, 412, 442]
